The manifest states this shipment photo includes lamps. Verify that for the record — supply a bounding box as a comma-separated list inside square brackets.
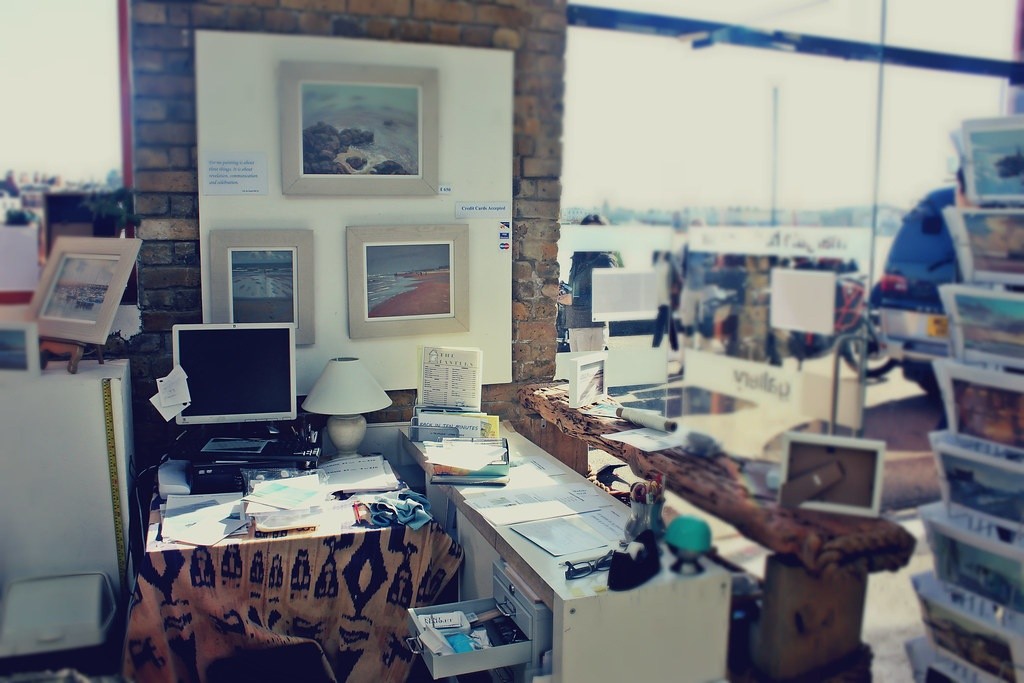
[301, 358, 393, 460]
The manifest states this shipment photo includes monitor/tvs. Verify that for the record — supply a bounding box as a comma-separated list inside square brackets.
[171, 323, 297, 442]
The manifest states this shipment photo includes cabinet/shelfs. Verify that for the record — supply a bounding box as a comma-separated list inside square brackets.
[398, 421, 732, 683]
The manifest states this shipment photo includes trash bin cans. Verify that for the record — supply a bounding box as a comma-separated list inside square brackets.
[0, 570, 117, 675]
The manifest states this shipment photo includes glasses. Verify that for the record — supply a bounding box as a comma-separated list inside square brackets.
[564, 549, 614, 579]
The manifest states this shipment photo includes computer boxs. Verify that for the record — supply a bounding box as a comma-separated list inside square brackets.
[192, 436, 321, 495]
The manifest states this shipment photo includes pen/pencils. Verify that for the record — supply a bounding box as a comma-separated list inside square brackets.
[291, 423, 318, 444]
[630, 471, 666, 504]
[353, 502, 361, 525]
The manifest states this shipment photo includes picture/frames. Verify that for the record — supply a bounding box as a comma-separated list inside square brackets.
[279, 59, 440, 195]
[209, 229, 316, 345]
[347, 226, 471, 339]
[26, 235, 142, 345]
[779, 432, 886, 518]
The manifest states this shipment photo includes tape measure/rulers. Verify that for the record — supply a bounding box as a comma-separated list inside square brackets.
[98, 374, 130, 620]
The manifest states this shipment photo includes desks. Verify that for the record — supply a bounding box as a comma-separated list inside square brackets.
[120, 451, 464, 683]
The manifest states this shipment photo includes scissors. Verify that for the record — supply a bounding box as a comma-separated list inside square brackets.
[634, 479, 660, 506]
